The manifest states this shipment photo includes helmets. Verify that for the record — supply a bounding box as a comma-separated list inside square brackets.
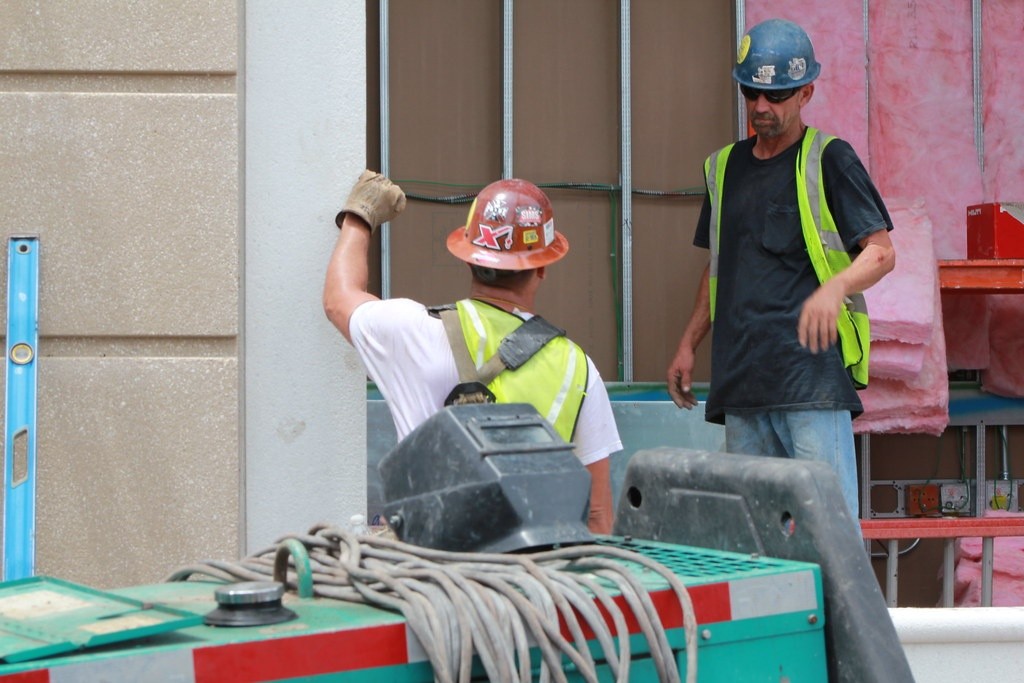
[446, 178, 569, 272]
[732, 17, 821, 89]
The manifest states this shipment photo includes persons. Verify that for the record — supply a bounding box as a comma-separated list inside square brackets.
[324, 169, 614, 537]
[666, 16, 895, 545]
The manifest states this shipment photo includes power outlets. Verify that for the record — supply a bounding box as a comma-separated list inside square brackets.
[985, 479, 1018, 514]
[906, 484, 939, 516]
[941, 482, 971, 514]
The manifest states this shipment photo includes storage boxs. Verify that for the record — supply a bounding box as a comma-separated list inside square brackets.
[966, 201, 1024, 259]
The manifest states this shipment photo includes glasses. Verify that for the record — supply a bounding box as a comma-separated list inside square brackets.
[740, 82, 803, 103]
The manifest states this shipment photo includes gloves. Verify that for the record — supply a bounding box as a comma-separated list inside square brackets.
[335, 168, 408, 237]
